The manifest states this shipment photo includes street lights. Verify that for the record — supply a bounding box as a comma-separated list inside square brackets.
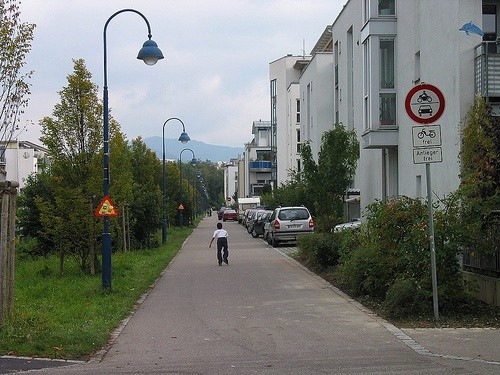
[100, 8, 165, 295]
[162, 117, 191, 246]
[179, 148, 198, 226]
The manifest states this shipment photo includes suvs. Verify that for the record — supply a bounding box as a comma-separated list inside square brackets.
[265, 205, 315, 248]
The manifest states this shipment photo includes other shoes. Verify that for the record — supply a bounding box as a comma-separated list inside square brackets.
[224, 259, 228, 265]
[219, 259, 222, 266]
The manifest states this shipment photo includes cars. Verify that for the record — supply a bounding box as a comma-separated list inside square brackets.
[217, 204, 295, 238]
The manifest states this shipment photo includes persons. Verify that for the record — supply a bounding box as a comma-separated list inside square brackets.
[209, 223, 228, 266]
